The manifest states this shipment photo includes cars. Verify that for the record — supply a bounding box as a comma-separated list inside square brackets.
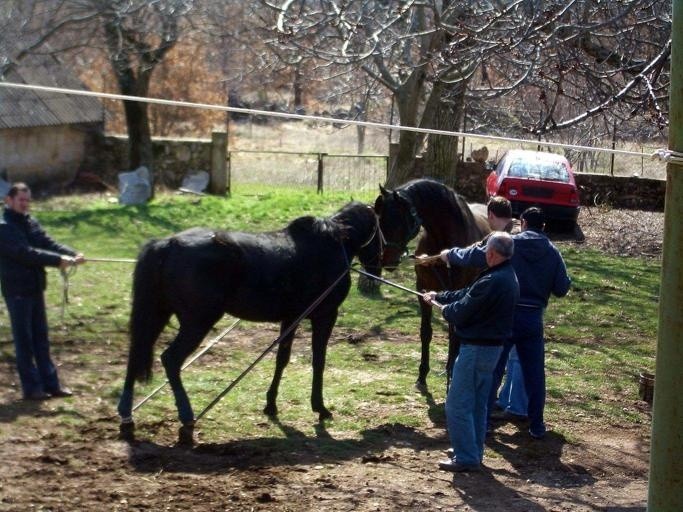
[483, 149, 581, 228]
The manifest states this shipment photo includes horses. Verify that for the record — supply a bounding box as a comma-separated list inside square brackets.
[375, 178, 494, 390]
[116, 197, 384, 443]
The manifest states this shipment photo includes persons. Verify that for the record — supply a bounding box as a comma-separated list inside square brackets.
[421, 230, 519, 473]
[439, 206, 571, 440]
[0, 180, 83, 401]
[478, 194, 531, 425]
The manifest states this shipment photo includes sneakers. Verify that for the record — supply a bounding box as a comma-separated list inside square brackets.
[24, 388, 72, 400]
[438, 449, 482, 472]
[489, 402, 545, 439]
